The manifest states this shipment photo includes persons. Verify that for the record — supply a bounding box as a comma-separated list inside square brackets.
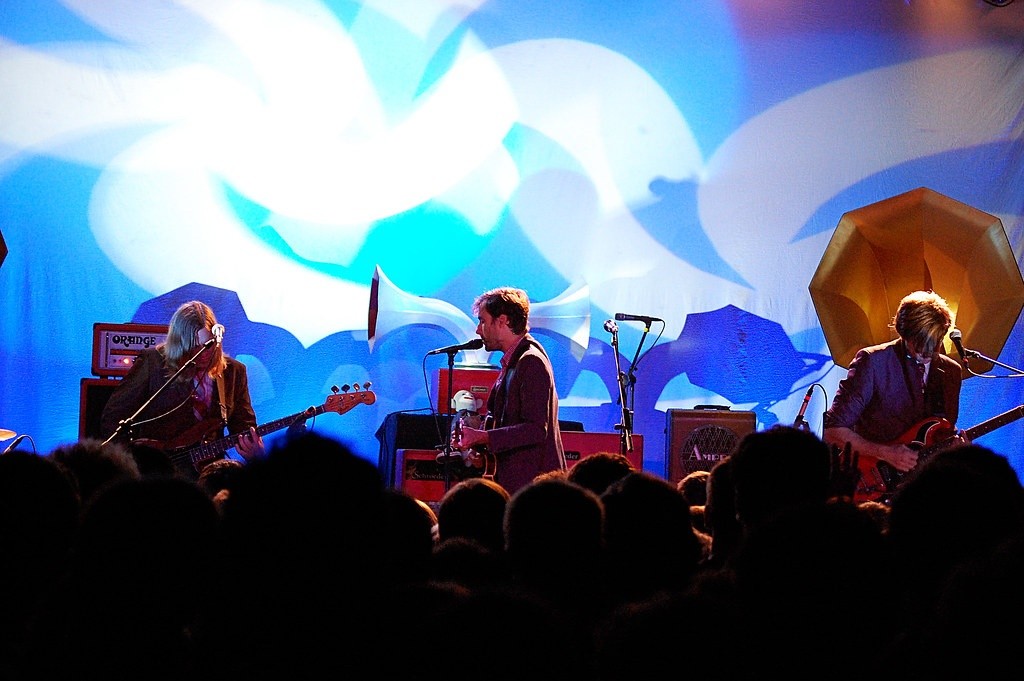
[101, 300, 264, 475]
[822, 292, 963, 506]
[0, 424, 1024, 681]
[436, 389, 483, 464]
[450, 289, 568, 493]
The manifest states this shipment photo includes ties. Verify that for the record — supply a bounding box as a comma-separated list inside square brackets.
[917, 363, 925, 391]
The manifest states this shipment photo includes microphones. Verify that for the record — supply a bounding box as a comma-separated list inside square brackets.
[212, 324, 225, 342]
[4, 435, 24, 453]
[604, 319, 618, 333]
[615, 313, 662, 322]
[950, 329, 969, 369]
[794, 386, 814, 427]
[427, 339, 483, 355]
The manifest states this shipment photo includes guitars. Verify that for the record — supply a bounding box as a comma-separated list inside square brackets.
[450, 415, 498, 480]
[131, 380, 377, 487]
[849, 405, 1024, 503]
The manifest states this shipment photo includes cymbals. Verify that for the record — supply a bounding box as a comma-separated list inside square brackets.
[0, 428, 17, 441]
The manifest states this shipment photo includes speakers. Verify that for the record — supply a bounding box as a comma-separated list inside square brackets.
[394, 366, 643, 517]
[78, 378, 127, 439]
[664, 408, 756, 484]
